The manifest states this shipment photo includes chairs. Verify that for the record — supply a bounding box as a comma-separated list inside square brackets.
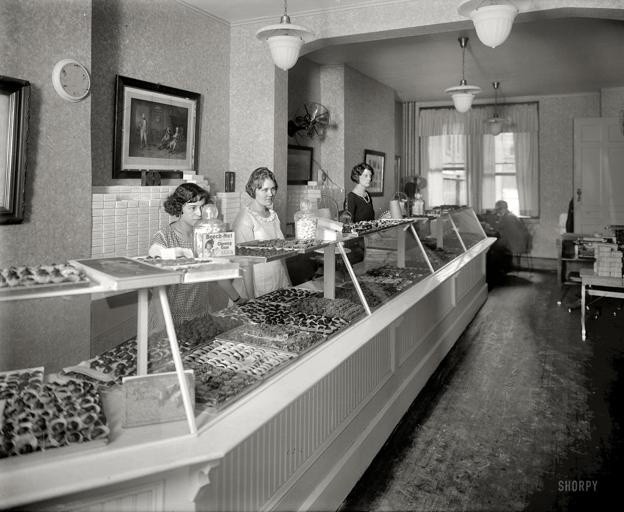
[504, 215, 536, 284]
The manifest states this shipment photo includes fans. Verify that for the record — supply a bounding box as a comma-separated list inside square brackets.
[288, 100, 334, 140]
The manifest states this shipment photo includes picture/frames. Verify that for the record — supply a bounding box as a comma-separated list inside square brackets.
[0, 75, 31, 225]
[107, 76, 202, 177]
[363, 149, 386, 197]
[287, 144, 313, 186]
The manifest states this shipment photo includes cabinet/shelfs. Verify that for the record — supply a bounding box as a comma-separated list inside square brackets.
[0, 201, 496, 511]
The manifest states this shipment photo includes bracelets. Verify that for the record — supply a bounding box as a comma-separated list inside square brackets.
[231, 294, 241, 303]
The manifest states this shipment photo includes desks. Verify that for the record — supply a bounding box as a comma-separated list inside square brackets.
[577, 267, 624, 340]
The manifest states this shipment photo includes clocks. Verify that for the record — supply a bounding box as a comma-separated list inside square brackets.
[51, 56, 94, 105]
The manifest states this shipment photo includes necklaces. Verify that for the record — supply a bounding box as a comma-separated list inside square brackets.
[359, 189, 371, 203]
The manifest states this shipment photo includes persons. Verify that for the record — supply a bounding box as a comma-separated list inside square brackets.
[168, 126, 182, 154]
[158, 125, 170, 149]
[229, 167, 292, 294]
[149, 183, 248, 329]
[482, 199, 530, 252]
[137, 111, 148, 152]
[343, 163, 374, 221]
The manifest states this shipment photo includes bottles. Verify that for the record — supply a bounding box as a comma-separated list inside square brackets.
[192, 204, 227, 259]
[293, 188, 338, 241]
[411, 193, 424, 217]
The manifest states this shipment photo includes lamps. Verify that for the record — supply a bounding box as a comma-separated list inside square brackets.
[485, 77, 507, 137]
[444, 32, 480, 112]
[455, 0, 523, 50]
[256, 0, 311, 71]
